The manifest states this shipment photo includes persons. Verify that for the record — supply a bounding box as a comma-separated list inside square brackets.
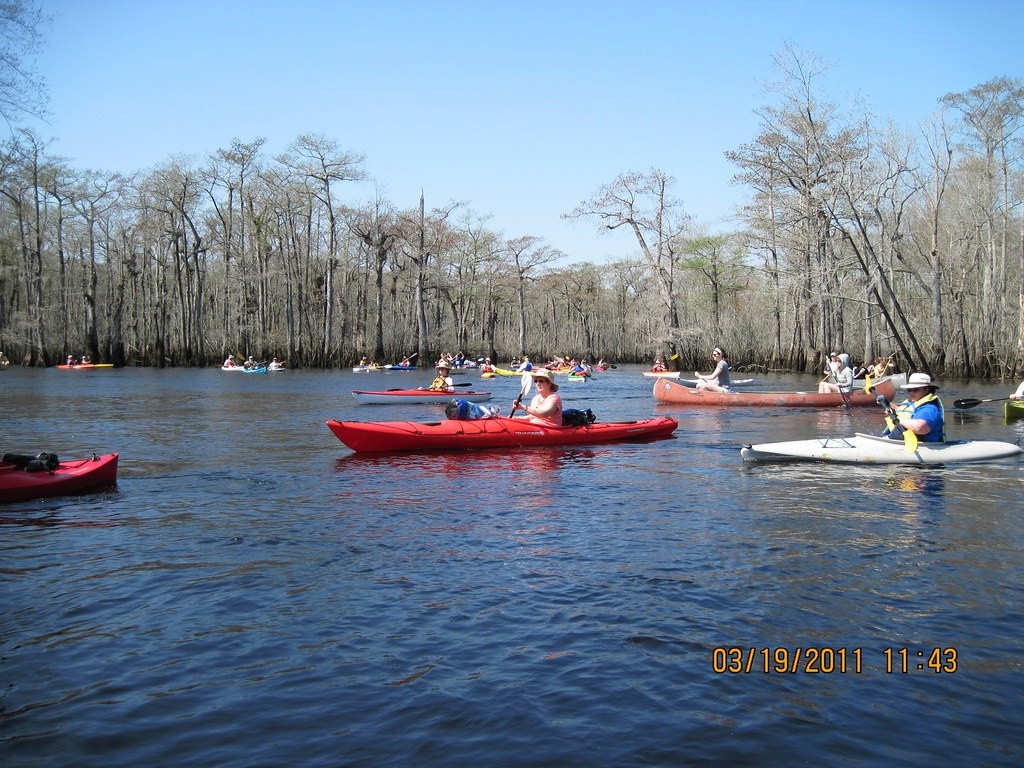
[481, 356, 496, 373]
[82, 356, 91, 364]
[819, 351, 896, 393]
[545, 355, 607, 375]
[511, 355, 532, 372]
[653, 359, 667, 372]
[67, 355, 75, 367]
[435, 351, 477, 369]
[876, 372, 946, 442]
[360, 357, 378, 368]
[224, 355, 236, 368]
[399, 356, 409, 367]
[418, 362, 455, 393]
[1009, 380, 1024, 401]
[0, 352, 9, 364]
[269, 357, 281, 368]
[244, 356, 258, 370]
[507, 368, 562, 427]
[694, 346, 731, 393]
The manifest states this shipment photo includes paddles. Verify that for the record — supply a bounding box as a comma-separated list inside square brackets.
[954, 395, 1024, 409]
[864, 373, 917, 454]
[566, 355, 575, 363]
[509, 372, 532, 419]
[87, 354, 91, 364]
[268, 360, 288, 370]
[827, 361, 851, 409]
[69, 359, 78, 368]
[816, 374, 831, 385]
[510, 351, 538, 377]
[695, 372, 709, 384]
[430, 354, 449, 367]
[577, 363, 598, 380]
[400, 353, 418, 366]
[463, 357, 484, 369]
[237, 352, 258, 368]
[875, 357, 892, 383]
[387, 382, 473, 391]
[650, 354, 679, 373]
[450, 352, 461, 362]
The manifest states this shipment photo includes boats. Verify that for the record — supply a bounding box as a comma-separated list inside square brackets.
[221, 365, 285, 373]
[326, 416, 678, 452]
[353, 365, 617, 383]
[653, 376, 896, 408]
[351, 389, 491, 404]
[1005, 401, 1024, 419]
[828, 372, 910, 390]
[676, 377, 754, 388]
[740, 432, 1024, 463]
[0, 362, 114, 370]
[643, 371, 680, 378]
[0, 452, 119, 501]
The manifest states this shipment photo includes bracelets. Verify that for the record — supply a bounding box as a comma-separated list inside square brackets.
[883, 399, 890, 407]
[525, 405, 528, 411]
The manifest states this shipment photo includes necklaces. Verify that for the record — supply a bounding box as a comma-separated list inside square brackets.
[539, 392, 549, 402]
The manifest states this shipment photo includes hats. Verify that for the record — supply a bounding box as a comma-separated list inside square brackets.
[830, 352, 838, 357]
[531, 368, 559, 392]
[485, 357, 491, 362]
[436, 361, 452, 370]
[900, 373, 940, 390]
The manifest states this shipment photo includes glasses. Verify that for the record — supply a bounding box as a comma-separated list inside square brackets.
[438, 368, 445, 370]
[711, 353, 719, 357]
[533, 379, 546, 384]
[835, 360, 841, 363]
[906, 387, 924, 393]
